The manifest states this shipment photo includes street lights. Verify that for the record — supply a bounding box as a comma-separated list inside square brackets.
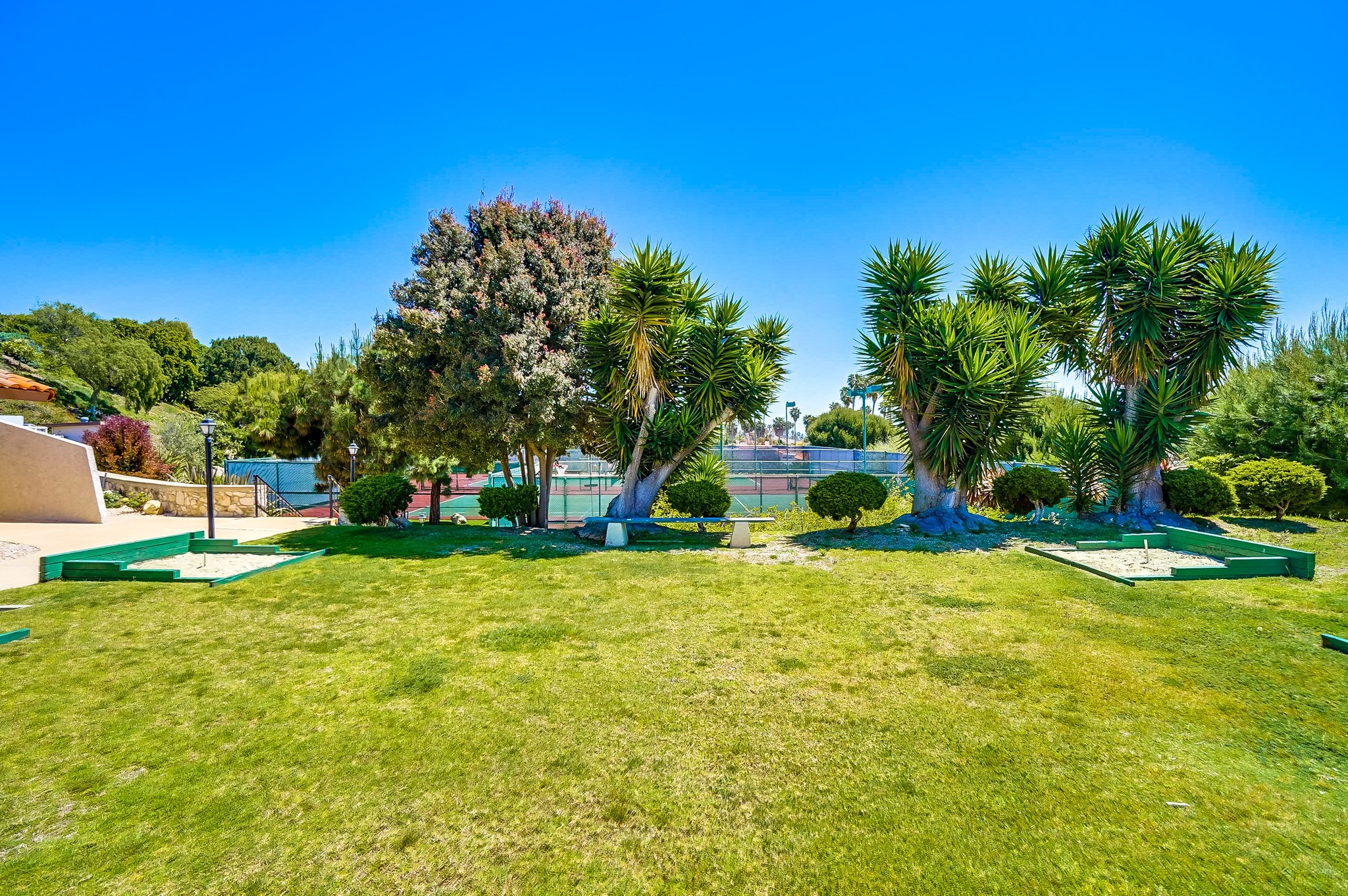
[347, 440, 360, 482]
[786, 401, 796, 489]
[198, 417, 218, 538]
[847, 384, 885, 473]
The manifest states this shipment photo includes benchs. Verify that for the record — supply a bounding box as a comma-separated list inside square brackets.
[583, 517, 775, 547]
